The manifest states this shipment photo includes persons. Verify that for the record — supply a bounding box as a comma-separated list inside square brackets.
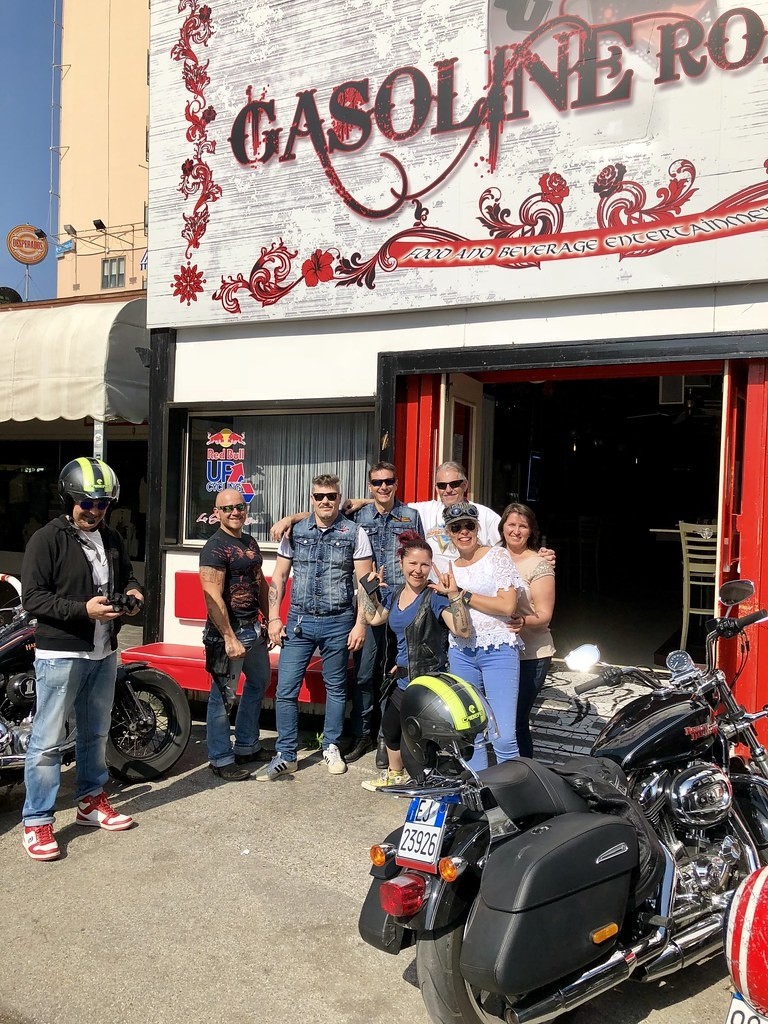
[342, 462, 556, 584]
[255, 474, 374, 780]
[21, 456, 145, 860]
[497, 504, 556, 758]
[434, 502, 524, 770]
[271, 462, 425, 769]
[361, 531, 471, 791]
[199, 489, 277, 781]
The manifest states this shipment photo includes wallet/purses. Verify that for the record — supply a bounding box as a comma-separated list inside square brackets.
[359, 573, 380, 595]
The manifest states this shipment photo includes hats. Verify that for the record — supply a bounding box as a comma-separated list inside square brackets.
[443, 504, 479, 525]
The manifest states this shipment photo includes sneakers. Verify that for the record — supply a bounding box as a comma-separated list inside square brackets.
[24, 824, 60, 860]
[256, 752, 297, 781]
[361, 766, 405, 791]
[76, 792, 133, 831]
[323, 744, 346, 774]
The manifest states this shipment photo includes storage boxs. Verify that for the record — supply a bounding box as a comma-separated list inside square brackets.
[461, 812, 638, 997]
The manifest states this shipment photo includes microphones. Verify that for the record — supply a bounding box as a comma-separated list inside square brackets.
[77, 518, 95, 524]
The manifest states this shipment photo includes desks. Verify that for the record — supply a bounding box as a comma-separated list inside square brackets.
[650, 525, 680, 543]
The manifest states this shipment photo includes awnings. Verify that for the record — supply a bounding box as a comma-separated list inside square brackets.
[0, 298, 150, 461]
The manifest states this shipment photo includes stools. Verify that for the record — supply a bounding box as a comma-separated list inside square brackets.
[571, 511, 607, 593]
[677, 519, 720, 652]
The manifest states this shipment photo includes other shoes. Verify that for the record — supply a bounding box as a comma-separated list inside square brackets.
[235, 747, 276, 763]
[344, 736, 373, 762]
[209, 763, 249, 780]
[376, 738, 390, 768]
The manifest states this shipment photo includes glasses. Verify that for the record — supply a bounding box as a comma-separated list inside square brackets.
[369, 478, 395, 486]
[80, 501, 111, 509]
[217, 503, 248, 512]
[450, 508, 479, 516]
[313, 493, 340, 500]
[451, 521, 475, 532]
[435, 480, 464, 489]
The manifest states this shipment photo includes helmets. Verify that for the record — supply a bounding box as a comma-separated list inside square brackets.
[58, 457, 120, 502]
[400, 672, 490, 765]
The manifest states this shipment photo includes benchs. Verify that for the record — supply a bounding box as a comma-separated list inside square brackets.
[119, 643, 352, 717]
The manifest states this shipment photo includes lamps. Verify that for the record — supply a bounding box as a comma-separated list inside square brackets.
[34, 229, 48, 238]
[93, 219, 106, 230]
[64, 224, 75, 235]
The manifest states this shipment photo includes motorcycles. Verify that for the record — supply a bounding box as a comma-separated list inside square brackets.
[358, 581, 768, 1024]
[0, 574, 190, 811]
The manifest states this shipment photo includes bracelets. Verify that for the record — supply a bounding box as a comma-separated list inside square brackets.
[522, 617, 525, 626]
[449, 595, 460, 602]
[268, 617, 280, 623]
[458, 588, 472, 603]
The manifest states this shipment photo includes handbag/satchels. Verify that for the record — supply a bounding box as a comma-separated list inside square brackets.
[206, 643, 230, 677]
